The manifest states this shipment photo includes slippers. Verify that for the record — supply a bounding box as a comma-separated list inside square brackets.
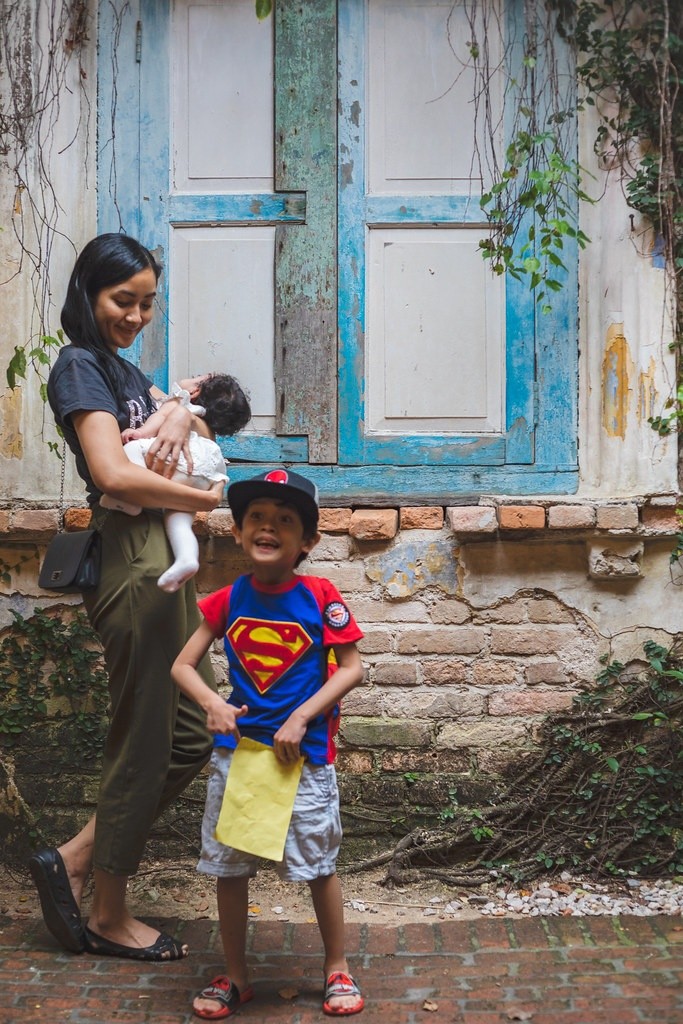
[320, 972, 365, 1015]
[192, 975, 255, 1018]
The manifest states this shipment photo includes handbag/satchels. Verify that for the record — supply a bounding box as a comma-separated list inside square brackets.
[37, 532, 105, 592]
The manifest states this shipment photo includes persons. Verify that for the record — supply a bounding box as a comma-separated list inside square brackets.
[24, 229, 215, 961]
[99, 370, 255, 591]
[168, 464, 367, 1016]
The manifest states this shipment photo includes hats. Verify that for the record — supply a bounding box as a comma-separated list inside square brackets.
[227, 470, 320, 536]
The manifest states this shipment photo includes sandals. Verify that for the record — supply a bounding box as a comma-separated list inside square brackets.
[83, 923, 190, 962]
[30, 848, 89, 955]
